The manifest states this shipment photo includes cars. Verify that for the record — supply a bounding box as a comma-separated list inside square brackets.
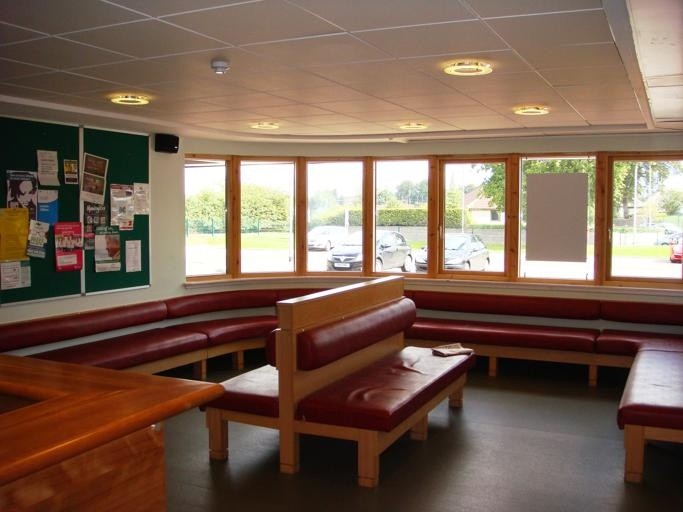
[306, 220, 350, 254]
[327, 229, 413, 275]
[669, 233, 683, 261]
[418, 225, 490, 273]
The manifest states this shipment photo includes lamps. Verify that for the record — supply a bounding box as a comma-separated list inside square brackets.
[400, 123, 428, 130]
[512, 103, 550, 116]
[440, 57, 493, 76]
[250, 121, 279, 130]
[109, 95, 150, 105]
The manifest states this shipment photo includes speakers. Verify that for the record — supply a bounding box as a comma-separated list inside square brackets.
[154, 132, 180, 154]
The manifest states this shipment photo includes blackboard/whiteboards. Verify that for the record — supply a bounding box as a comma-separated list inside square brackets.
[81, 124, 153, 297]
[0, 114, 84, 307]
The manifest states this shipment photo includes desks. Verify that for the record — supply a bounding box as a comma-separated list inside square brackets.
[0, 353, 226, 512]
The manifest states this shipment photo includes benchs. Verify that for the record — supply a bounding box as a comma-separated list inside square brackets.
[0, 300, 209, 381]
[415, 290, 600, 387]
[164, 288, 415, 381]
[596, 300, 683, 484]
